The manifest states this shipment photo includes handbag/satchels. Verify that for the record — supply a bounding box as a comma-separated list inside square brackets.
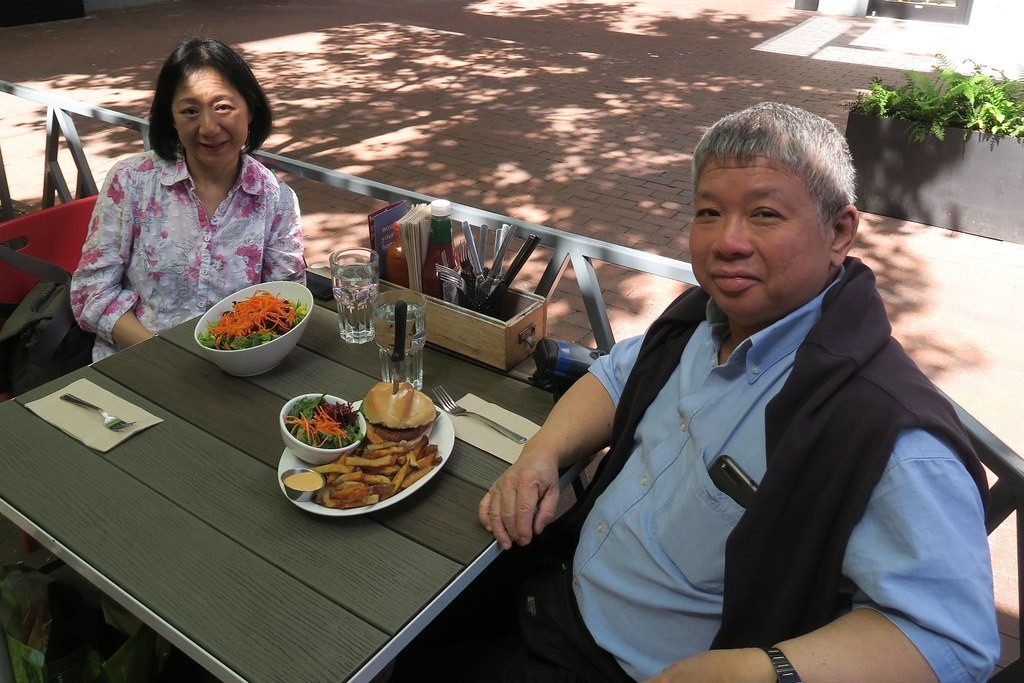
[0, 282, 95, 397]
[0, 559, 157, 683]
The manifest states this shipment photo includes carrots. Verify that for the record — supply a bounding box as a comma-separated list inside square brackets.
[211, 290, 297, 349]
[284, 406, 351, 448]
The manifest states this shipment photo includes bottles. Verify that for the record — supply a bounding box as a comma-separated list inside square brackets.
[386, 221, 409, 290]
[421, 199, 460, 299]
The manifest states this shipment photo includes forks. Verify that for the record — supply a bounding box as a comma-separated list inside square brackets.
[460, 239, 474, 280]
[435, 263, 468, 305]
[59, 393, 137, 431]
[432, 386, 527, 444]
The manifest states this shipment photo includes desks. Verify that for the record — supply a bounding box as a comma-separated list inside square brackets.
[0, 264, 603, 683]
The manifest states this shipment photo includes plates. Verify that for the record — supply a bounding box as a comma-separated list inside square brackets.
[277, 399, 455, 516]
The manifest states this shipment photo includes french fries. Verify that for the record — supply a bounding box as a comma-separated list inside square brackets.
[310, 436, 442, 510]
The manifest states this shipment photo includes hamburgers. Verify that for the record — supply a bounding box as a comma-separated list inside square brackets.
[364, 382, 437, 446]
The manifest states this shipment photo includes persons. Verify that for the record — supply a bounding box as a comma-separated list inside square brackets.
[384, 101, 1001, 683]
[69, 38, 305, 365]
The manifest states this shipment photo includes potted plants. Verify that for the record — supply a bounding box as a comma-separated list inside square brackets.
[846, 50, 1024, 245]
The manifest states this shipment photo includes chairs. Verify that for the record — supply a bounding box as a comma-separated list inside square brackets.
[933, 380, 1023, 683]
[0, 195, 102, 404]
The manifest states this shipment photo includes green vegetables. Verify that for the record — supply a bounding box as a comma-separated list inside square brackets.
[198, 298, 307, 350]
[286, 393, 363, 448]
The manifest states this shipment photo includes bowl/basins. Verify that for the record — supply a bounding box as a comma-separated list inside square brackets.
[194, 280, 314, 376]
[279, 393, 367, 463]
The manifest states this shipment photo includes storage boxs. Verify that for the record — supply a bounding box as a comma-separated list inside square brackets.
[378, 277, 546, 371]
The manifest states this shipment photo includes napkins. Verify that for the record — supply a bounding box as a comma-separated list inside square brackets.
[25, 377, 165, 453]
[442, 393, 541, 465]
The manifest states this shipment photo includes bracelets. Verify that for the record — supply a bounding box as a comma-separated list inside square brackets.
[762, 647, 803, 683]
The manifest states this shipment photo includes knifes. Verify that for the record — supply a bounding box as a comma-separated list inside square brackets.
[461, 218, 542, 308]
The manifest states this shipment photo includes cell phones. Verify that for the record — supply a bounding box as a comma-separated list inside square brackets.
[708, 454, 761, 508]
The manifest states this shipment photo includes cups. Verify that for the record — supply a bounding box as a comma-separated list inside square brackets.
[329, 246, 380, 344]
[282, 468, 326, 506]
[370, 290, 427, 393]
